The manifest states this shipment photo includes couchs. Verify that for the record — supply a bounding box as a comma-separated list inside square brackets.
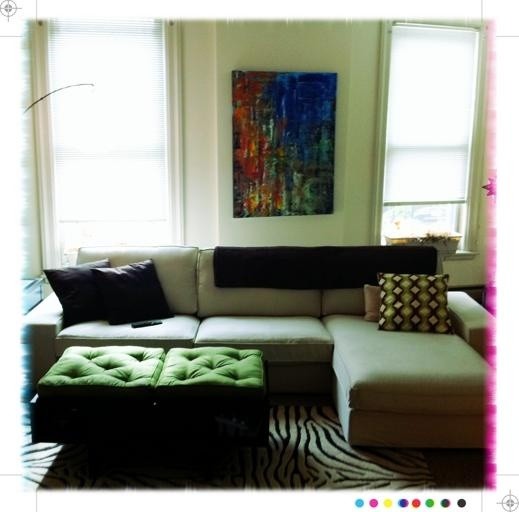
[23, 243, 496, 451]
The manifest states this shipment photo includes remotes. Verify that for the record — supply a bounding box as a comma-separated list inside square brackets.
[132, 320, 162, 327]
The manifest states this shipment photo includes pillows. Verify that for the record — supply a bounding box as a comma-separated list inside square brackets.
[40, 257, 175, 332]
[360, 271, 453, 334]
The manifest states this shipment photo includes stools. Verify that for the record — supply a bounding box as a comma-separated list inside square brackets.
[31, 346, 270, 450]
